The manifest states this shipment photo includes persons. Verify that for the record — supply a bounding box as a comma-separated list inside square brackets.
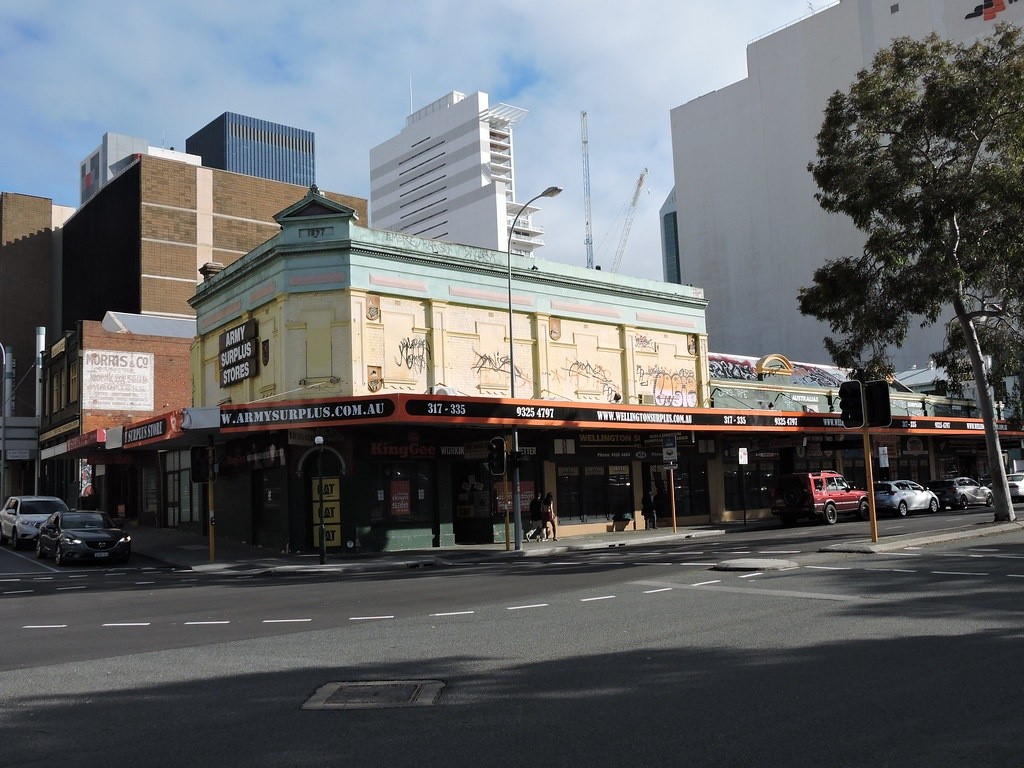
[537, 492, 559, 541]
[85, 487, 100, 511]
[641, 492, 658, 530]
[526, 493, 548, 542]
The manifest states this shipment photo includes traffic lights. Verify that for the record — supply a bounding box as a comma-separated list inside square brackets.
[487, 436, 505, 477]
[838, 381, 865, 429]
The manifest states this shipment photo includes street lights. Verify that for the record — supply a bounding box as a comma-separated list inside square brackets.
[506, 187, 565, 551]
[314, 435, 325, 564]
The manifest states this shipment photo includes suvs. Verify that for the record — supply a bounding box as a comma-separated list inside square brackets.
[770, 469, 868, 525]
[0, 495, 68, 550]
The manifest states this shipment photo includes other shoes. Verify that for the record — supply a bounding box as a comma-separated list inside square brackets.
[536, 535, 540, 542]
[526, 534, 530, 543]
[645, 528, 650, 530]
[542, 538, 548, 541]
[553, 538, 559, 541]
[653, 527, 657, 529]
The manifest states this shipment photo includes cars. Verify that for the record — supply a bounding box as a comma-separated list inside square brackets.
[987, 473, 1024, 503]
[35, 508, 132, 568]
[873, 480, 940, 518]
[926, 476, 995, 510]
